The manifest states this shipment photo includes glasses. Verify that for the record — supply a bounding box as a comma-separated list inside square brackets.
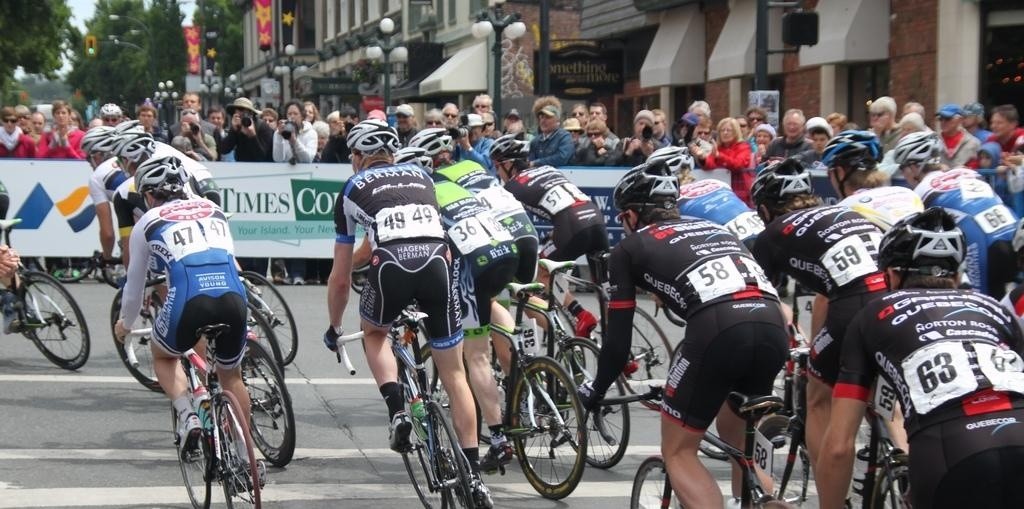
[586, 132, 604, 137]
[444, 113, 458, 118]
[426, 120, 442, 125]
[872, 111, 890, 118]
[571, 111, 586, 118]
[181, 108, 197, 115]
[538, 114, 555, 120]
[475, 105, 490, 109]
[697, 131, 709, 135]
[655, 121, 660, 125]
[590, 112, 601, 115]
[486, 122, 494, 127]
[103, 118, 118, 122]
[397, 114, 409, 119]
[1, 118, 20, 124]
[741, 124, 748, 127]
[938, 116, 954, 122]
[263, 118, 276, 124]
[748, 116, 765, 122]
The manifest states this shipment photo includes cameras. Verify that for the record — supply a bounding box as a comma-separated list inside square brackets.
[190, 121, 200, 134]
[449, 125, 470, 138]
[240, 116, 253, 127]
[342, 121, 355, 131]
[280, 120, 295, 139]
[759, 154, 770, 165]
[641, 125, 653, 140]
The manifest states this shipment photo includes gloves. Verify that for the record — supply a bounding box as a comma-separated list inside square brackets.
[323, 325, 341, 352]
[577, 383, 603, 411]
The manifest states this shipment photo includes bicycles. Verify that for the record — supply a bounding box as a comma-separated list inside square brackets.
[563, 248, 672, 411]
[352, 265, 587, 500]
[124, 328, 260, 507]
[97, 257, 285, 393]
[336, 309, 483, 509]
[239, 271, 299, 366]
[594, 384, 794, 507]
[113, 309, 296, 467]
[754, 347, 916, 508]
[511, 259, 630, 470]
[0, 218, 90, 370]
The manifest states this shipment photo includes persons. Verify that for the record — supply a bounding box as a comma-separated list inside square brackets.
[901, 102, 935, 132]
[816, 206, 1024, 509]
[89, 117, 101, 131]
[487, 134, 638, 384]
[892, 131, 1022, 296]
[175, 109, 218, 161]
[320, 107, 359, 163]
[221, 97, 275, 161]
[839, 122, 861, 131]
[15, 106, 31, 130]
[822, 128, 925, 224]
[755, 123, 777, 147]
[273, 99, 318, 163]
[326, 111, 341, 132]
[732, 114, 749, 134]
[696, 116, 712, 140]
[27, 112, 48, 159]
[962, 102, 993, 138]
[932, 104, 982, 170]
[532, 95, 561, 113]
[677, 113, 713, 161]
[825, 112, 847, 134]
[114, 158, 254, 495]
[421, 107, 444, 130]
[324, 117, 494, 509]
[649, 147, 801, 369]
[981, 103, 1024, 168]
[606, 110, 665, 166]
[111, 131, 213, 390]
[68, 109, 84, 131]
[101, 103, 122, 125]
[806, 114, 833, 156]
[394, 104, 421, 150]
[258, 106, 278, 132]
[300, 102, 322, 121]
[747, 108, 766, 129]
[688, 102, 711, 115]
[456, 114, 500, 175]
[392, 147, 519, 472]
[311, 122, 331, 163]
[746, 156, 909, 509]
[760, 109, 814, 162]
[81, 125, 250, 331]
[472, 96, 494, 115]
[688, 118, 754, 210]
[366, 110, 386, 125]
[578, 102, 622, 151]
[137, 105, 166, 145]
[442, 103, 459, 128]
[561, 115, 585, 166]
[171, 135, 209, 163]
[976, 141, 1000, 171]
[586, 159, 789, 509]
[0, 107, 26, 159]
[650, 110, 675, 148]
[502, 107, 537, 151]
[169, 92, 221, 162]
[571, 119, 614, 166]
[480, 115, 501, 135]
[897, 113, 927, 138]
[570, 103, 589, 128]
[869, 96, 898, 143]
[527, 107, 574, 169]
[1, 173, 16, 336]
[403, 128, 539, 438]
[39, 101, 87, 160]
[207, 103, 235, 162]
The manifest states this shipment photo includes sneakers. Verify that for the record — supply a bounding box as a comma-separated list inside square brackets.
[25, 263, 104, 284]
[477, 439, 515, 472]
[571, 310, 598, 338]
[386, 410, 415, 453]
[467, 473, 497, 508]
[620, 361, 640, 379]
[250, 272, 330, 286]
[178, 413, 202, 464]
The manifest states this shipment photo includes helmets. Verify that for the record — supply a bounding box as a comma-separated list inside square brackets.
[820, 130, 884, 168]
[893, 130, 942, 166]
[79, 125, 120, 157]
[750, 157, 814, 202]
[134, 154, 189, 195]
[409, 127, 455, 155]
[345, 119, 400, 154]
[878, 207, 968, 277]
[614, 159, 681, 214]
[489, 131, 532, 162]
[649, 147, 696, 175]
[392, 147, 434, 175]
[111, 133, 157, 162]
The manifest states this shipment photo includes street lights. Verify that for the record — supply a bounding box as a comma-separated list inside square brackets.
[200, 69, 219, 108]
[222, 72, 245, 102]
[154, 80, 178, 125]
[108, 13, 157, 81]
[198, 67, 222, 118]
[272, 39, 308, 113]
[154, 79, 179, 120]
[274, 45, 310, 100]
[107, 12, 157, 93]
[225, 73, 244, 100]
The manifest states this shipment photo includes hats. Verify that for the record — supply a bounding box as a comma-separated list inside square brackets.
[934, 104, 966, 121]
[804, 117, 835, 137]
[467, 112, 487, 128]
[503, 108, 524, 120]
[633, 109, 657, 124]
[751, 123, 779, 140]
[225, 98, 264, 117]
[680, 112, 701, 127]
[962, 100, 986, 119]
